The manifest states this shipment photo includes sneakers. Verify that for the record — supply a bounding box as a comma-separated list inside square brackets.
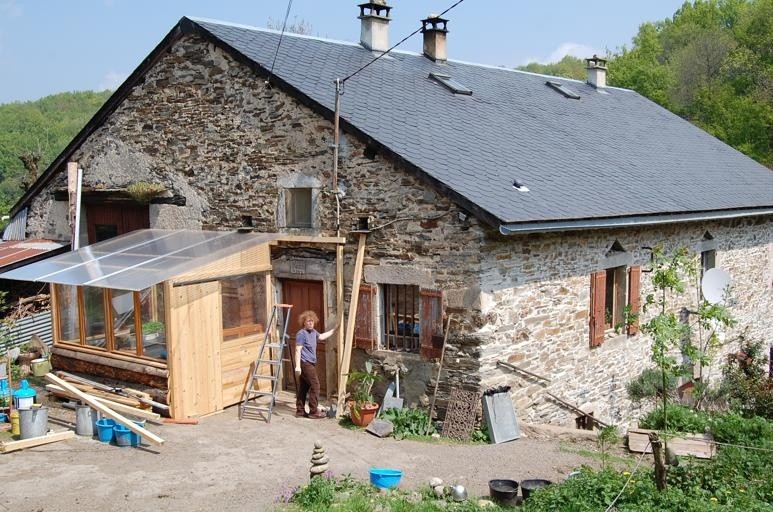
[308, 410, 327, 418]
[295, 410, 309, 418]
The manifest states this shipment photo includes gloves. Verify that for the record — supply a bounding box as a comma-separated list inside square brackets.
[295, 368, 302, 376]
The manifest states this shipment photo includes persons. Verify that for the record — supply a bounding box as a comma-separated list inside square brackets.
[293, 309, 340, 419]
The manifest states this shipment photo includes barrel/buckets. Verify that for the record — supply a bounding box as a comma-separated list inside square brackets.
[368, 467, 404, 491]
[75, 404, 103, 438]
[30, 358, 50, 378]
[10, 413, 20, 434]
[18, 406, 49, 440]
[520, 478, 555, 498]
[112, 422, 144, 447]
[489, 478, 519, 509]
[95, 417, 116, 442]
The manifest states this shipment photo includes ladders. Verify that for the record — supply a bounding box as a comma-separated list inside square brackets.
[426, 315, 464, 437]
[240, 304, 299, 423]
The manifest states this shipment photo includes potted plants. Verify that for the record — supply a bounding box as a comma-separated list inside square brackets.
[343, 370, 381, 426]
[142, 321, 162, 342]
